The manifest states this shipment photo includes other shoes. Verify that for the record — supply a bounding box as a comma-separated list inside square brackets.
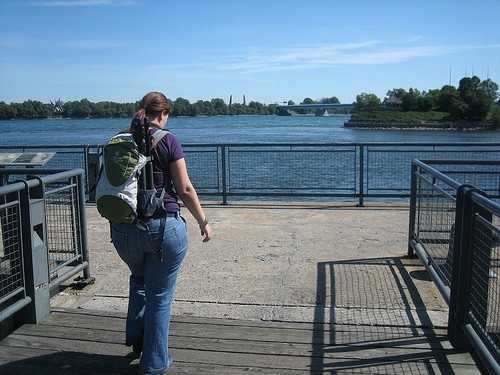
[132, 341, 142, 357]
[138, 354, 172, 375]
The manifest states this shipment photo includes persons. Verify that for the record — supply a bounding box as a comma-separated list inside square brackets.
[106, 91, 213, 374]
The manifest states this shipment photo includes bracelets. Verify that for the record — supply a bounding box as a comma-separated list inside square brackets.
[199, 217, 209, 228]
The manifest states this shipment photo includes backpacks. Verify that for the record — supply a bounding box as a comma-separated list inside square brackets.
[89, 128, 168, 225]
